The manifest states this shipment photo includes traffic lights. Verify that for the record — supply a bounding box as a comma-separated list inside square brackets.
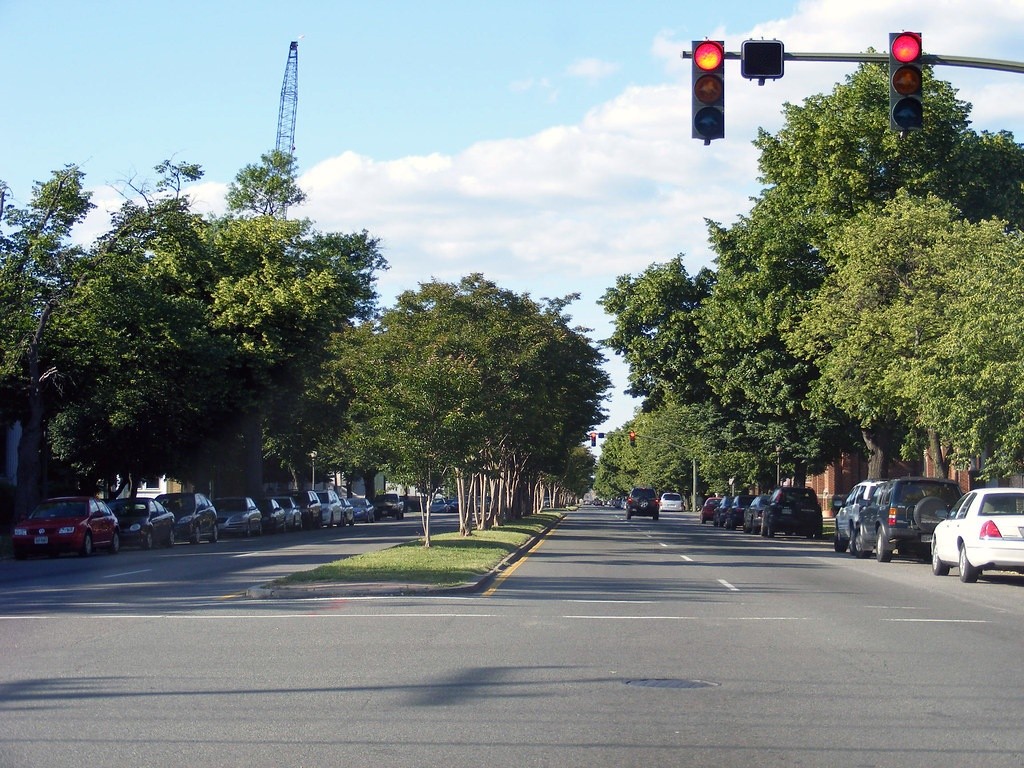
[889, 32, 923, 138]
[630, 431, 635, 446]
[591, 433, 596, 446]
[743, 38, 784, 82]
[692, 39, 724, 145]
[598, 433, 604, 438]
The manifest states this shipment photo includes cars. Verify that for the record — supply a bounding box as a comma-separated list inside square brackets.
[251, 496, 287, 534]
[315, 490, 342, 528]
[430, 496, 491, 513]
[155, 493, 219, 544]
[12, 496, 121, 561]
[712, 496, 735, 527]
[584, 497, 627, 510]
[108, 497, 176, 550]
[347, 498, 375, 522]
[743, 493, 772, 534]
[723, 495, 757, 530]
[211, 497, 262, 538]
[931, 488, 1024, 583]
[700, 497, 723, 524]
[287, 490, 323, 530]
[339, 497, 355, 527]
[274, 497, 304, 532]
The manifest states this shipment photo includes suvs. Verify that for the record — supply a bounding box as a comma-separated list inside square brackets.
[852, 476, 963, 562]
[761, 486, 823, 539]
[371, 493, 406, 520]
[626, 487, 661, 520]
[833, 479, 888, 552]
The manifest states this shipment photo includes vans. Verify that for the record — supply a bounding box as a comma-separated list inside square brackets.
[660, 492, 684, 512]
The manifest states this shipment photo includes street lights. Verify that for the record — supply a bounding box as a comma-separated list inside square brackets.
[775, 444, 783, 486]
[310, 448, 318, 491]
